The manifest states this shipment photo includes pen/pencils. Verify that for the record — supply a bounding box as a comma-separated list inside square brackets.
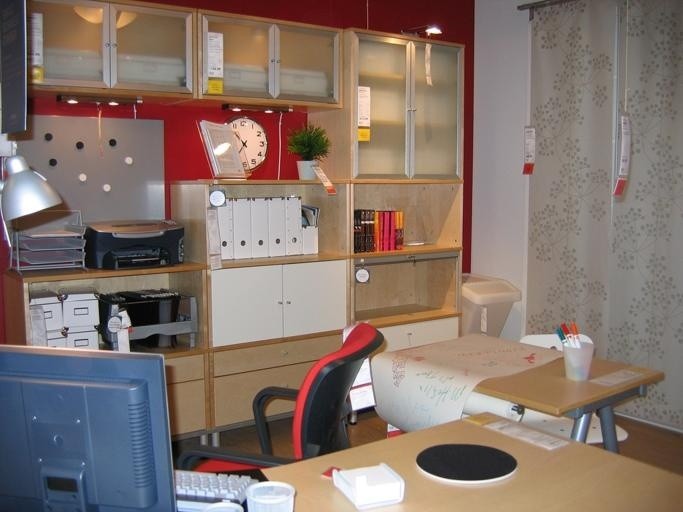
[557, 321, 587, 377]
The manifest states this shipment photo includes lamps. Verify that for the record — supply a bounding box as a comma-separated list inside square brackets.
[0, 152, 65, 231]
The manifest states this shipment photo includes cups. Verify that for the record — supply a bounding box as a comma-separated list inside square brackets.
[562, 342, 595, 380]
[204, 482, 296, 512]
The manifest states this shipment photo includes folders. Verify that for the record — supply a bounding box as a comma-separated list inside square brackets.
[220, 198, 304, 259]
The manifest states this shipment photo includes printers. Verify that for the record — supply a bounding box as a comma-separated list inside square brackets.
[65, 219, 185, 269]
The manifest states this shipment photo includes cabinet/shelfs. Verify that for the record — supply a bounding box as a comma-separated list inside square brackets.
[197, 9, 344, 111]
[2, 262, 206, 444]
[169, 181, 346, 447]
[348, 31, 465, 418]
[26, 0, 196, 99]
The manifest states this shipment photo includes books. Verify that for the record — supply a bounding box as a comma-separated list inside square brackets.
[352, 207, 404, 254]
[300, 205, 321, 227]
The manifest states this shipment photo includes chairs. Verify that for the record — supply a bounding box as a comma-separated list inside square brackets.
[177, 324, 384, 476]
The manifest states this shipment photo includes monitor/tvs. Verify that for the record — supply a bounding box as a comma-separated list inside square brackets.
[1, 344, 177, 511]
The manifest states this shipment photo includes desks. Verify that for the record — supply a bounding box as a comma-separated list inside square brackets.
[370, 331, 668, 459]
[173, 414, 682, 511]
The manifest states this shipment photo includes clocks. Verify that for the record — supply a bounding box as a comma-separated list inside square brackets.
[218, 114, 269, 174]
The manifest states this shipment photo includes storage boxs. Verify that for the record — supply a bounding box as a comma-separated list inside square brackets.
[29, 287, 184, 357]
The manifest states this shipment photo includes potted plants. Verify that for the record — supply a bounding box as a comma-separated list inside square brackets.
[285, 122, 333, 182]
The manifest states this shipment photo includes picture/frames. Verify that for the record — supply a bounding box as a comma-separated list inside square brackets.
[195, 120, 249, 180]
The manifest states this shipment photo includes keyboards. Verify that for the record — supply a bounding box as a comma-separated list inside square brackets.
[175, 469, 258, 503]
